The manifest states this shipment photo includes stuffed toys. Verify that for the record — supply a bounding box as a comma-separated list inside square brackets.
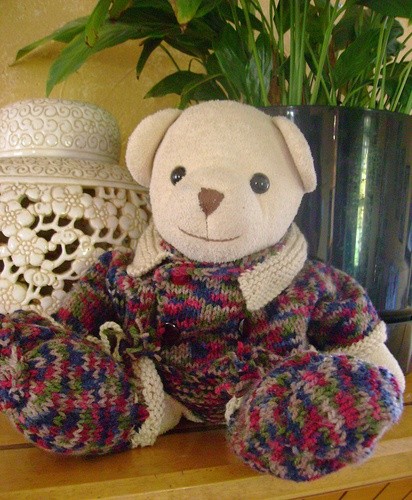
[1, 100, 405, 481]
